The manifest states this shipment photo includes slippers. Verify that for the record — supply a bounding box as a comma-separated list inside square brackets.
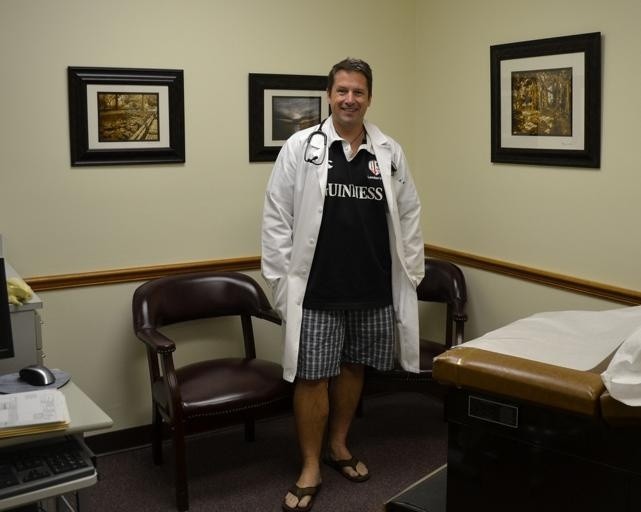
[322, 454, 371, 482]
[282, 482, 322, 512]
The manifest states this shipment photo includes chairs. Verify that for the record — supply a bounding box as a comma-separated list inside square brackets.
[352, 253, 465, 419]
[133, 271, 300, 512]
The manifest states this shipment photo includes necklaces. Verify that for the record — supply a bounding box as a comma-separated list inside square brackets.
[336, 128, 364, 145]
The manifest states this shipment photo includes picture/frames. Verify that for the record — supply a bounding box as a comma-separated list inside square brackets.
[248, 71, 333, 161]
[489, 30, 601, 168]
[67, 65, 186, 165]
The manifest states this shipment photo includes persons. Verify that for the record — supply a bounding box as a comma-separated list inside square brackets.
[261, 60, 425, 512]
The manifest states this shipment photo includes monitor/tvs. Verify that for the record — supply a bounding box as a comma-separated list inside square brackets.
[0, 258, 15, 361]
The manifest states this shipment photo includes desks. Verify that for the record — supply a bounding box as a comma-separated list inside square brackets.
[0, 366, 115, 510]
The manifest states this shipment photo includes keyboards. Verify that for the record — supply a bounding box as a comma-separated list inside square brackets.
[0, 437, 95, 499]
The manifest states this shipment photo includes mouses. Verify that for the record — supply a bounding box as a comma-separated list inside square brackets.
[19, 364, 55, 386]
